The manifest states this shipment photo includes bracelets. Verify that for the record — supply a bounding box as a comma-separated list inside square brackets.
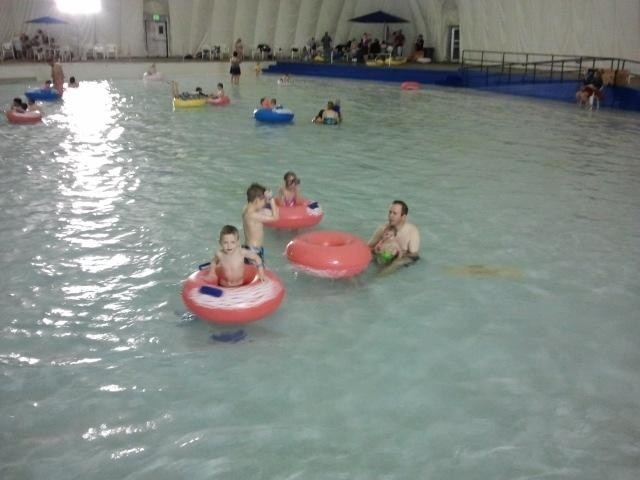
[257, 263, 263, 269]
[269, 196, 274, 200]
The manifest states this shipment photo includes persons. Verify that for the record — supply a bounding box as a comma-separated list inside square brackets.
[365, 200, 421, 276]
[277, 170, 302, 234]
[373, 226, 404, 267]
[242, 184, 276, 268]
[174, 28, 424, 125]
[203, 225, 269, 287]
[2, 29, 80, 112]
[150, 63, 158, 75]
[575, 71, 604, 107]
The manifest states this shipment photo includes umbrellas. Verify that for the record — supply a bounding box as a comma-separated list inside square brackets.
[27, 16, 68, 46]
[348, 10, 411, 49]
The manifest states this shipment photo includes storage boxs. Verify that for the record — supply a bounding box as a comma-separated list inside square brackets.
[588, 68, 614, 86]
[616, 69, 631, 85]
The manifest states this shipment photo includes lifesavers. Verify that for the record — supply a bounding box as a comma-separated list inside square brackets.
[209, 96, 229, 105]
[261, 196, 323, 228]
[25, 89, 60, 100]
[400, 82, 420, 90]
[286, 231, 372, 278]
[183, 263, 285, 323]
[173, 97, 206, 107]
[6, 109, 41, 123]
[376, 51, 431, 65]
[254, 108, 294, 122]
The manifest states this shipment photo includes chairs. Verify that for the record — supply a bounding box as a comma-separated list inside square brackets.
[1, 34, 121, 62]
[200, 41, 398, 63]
